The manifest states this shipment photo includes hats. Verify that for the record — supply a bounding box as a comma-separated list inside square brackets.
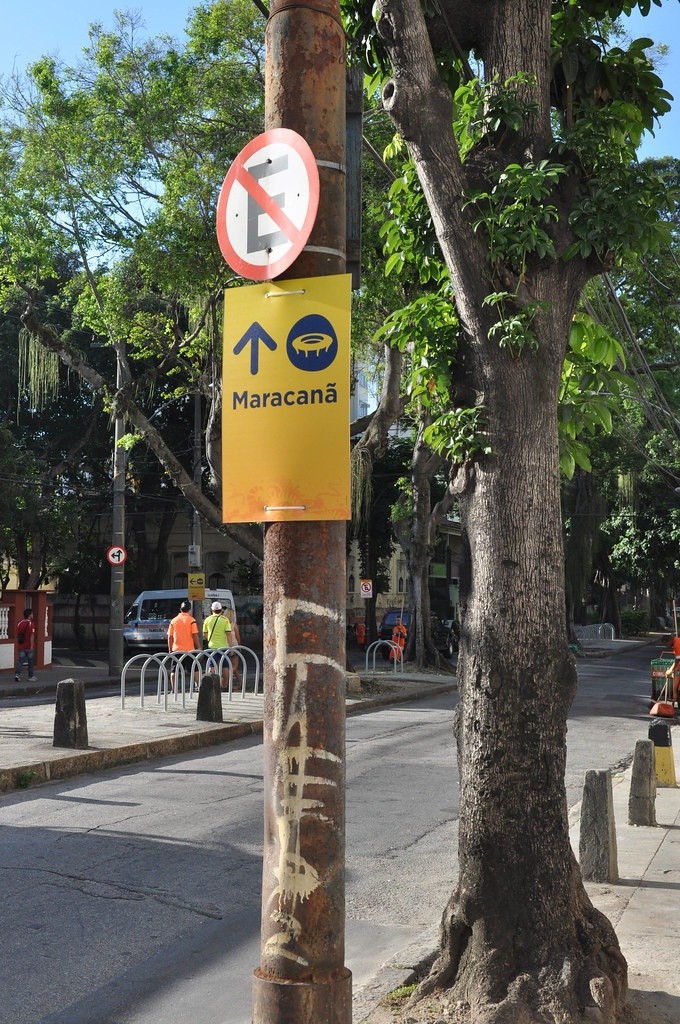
[181, 600, 191, 609]
[211, 602, 222, 610]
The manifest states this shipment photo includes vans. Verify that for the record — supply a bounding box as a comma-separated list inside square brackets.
[124, 588, 237, 656]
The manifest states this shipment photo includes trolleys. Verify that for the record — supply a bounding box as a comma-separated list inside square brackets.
[649, 651, 673, 709]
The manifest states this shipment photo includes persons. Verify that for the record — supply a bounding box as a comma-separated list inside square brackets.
[14, 608, 39, 682]
[203, 601, 243, 692]
[389, 615, 408, 665]
[166, 600, 201, 695]
[346, 620, 367, 654]
[661, 633, 680, 710]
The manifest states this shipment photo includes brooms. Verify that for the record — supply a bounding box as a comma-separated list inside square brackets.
[390, 596, 405, 666]
[647, 680, 675, 711]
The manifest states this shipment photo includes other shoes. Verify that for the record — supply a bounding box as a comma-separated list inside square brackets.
[196, 687, 199, 692]
[28, 676, 38, 682]
[222, 687, 234, 692]
[15, 674, 21, 681]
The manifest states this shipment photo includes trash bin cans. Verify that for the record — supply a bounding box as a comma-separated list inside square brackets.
[651, 657, 675, 701]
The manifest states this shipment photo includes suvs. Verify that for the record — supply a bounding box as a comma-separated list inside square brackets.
[378, 608, 457, 659]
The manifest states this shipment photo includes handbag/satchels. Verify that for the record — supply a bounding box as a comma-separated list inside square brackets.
[202, 639, 208, 659]
[18, 633, 25, 644]
